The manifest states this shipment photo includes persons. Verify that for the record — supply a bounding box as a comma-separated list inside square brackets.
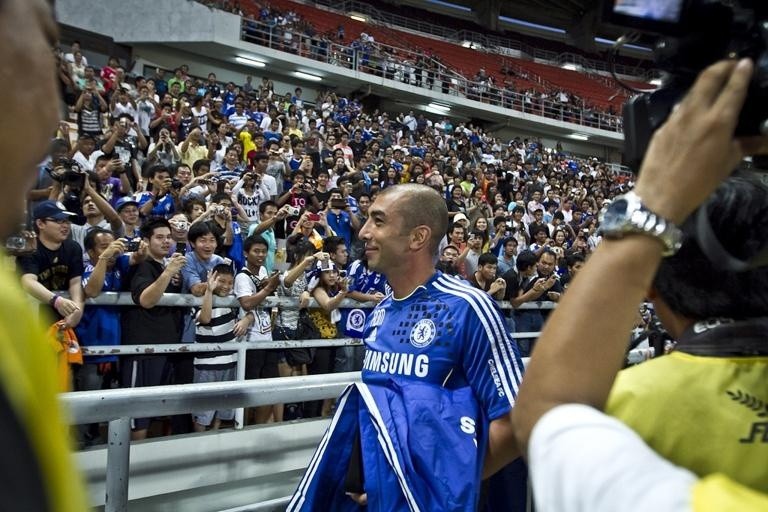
[600, 237, 766, 491]
[19, 2, 683, 443]
[351, 183, 528, 512]
[0, 1, 93, 510]
[511, 54, 768, 509]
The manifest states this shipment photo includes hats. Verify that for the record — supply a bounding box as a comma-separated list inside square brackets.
[452, 213, 471, 229]
[113, 196, 140, 212]
[31, 199, 79, 221]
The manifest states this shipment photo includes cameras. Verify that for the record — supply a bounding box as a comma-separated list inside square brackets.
[216, 205, 226, 220]
[299, 184, 306, 188]
[289, 208, 300, 216]
[171, 178, 182, 189]
[118, 89, 125, 95]
[339, 270, 347, 281]
[125, 241, 139, 252]
[249, 172, 257, 179]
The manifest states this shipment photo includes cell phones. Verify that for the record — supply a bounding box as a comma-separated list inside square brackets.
[176, 243, 186, 256]
[470, 234, 475, 239]
[347, 183, 353, 193]
[308, 214, 320, 221]
